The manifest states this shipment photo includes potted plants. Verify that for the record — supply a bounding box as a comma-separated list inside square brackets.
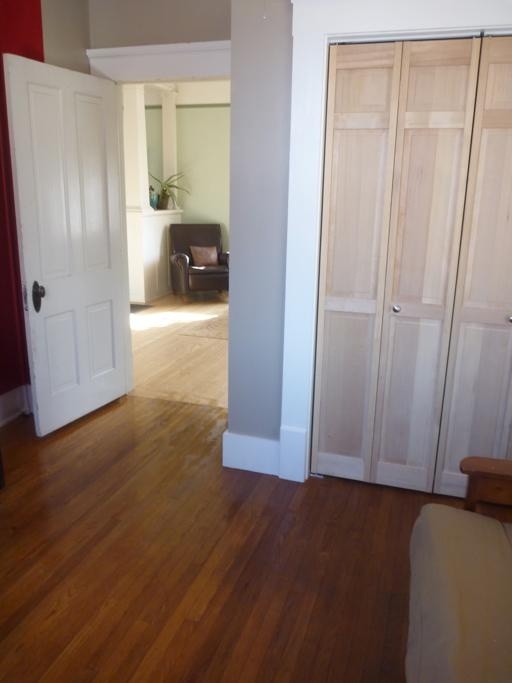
[148, 170, 192, 211]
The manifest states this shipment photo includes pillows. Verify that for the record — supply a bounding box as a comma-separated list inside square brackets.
[188, 244, 220, 267]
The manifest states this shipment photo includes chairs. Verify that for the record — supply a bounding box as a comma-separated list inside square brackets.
[168, 222, 230, 298]
